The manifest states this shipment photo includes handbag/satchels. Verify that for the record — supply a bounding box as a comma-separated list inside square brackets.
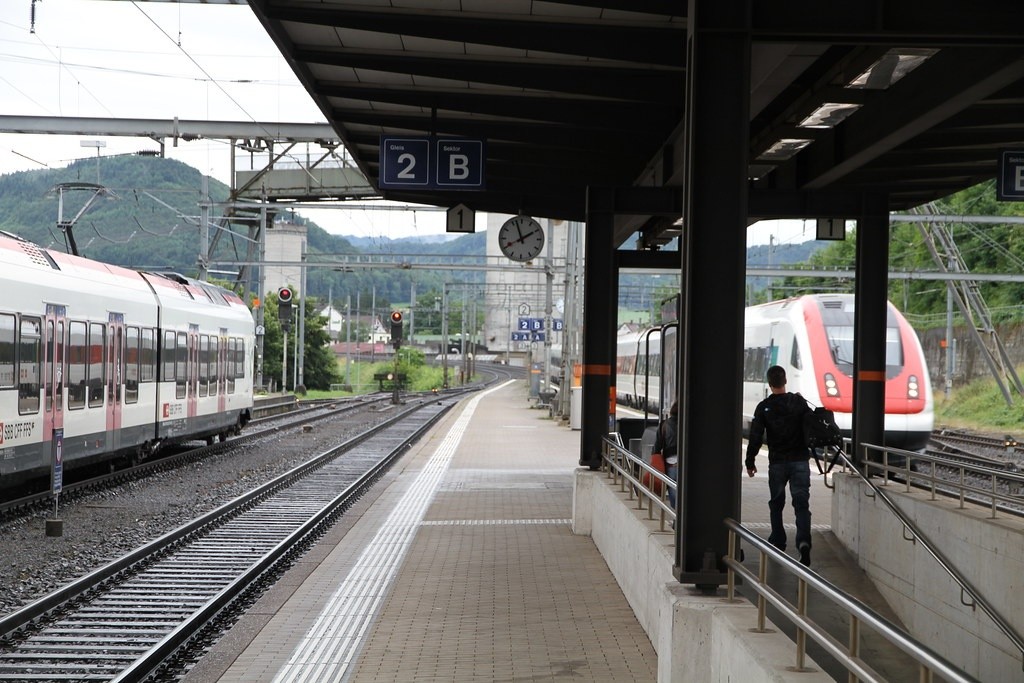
[802, 406, 844, 448]
[642, 453, 666, 494]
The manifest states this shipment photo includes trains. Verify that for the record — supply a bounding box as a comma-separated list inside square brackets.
[0, 230, 256, 498]
[551, 286, 935, 478]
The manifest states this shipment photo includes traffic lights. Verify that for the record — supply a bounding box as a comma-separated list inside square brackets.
[386, 373, 394, 380]
[390, 311, 403, 339]
[278, 287, 292, 319]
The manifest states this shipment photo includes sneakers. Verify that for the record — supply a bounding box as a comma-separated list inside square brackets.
[796, 541, 811, 567]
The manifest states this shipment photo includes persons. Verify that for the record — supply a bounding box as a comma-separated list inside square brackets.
[652, 400, 678, 525]
[745, 365, 820, 570]
